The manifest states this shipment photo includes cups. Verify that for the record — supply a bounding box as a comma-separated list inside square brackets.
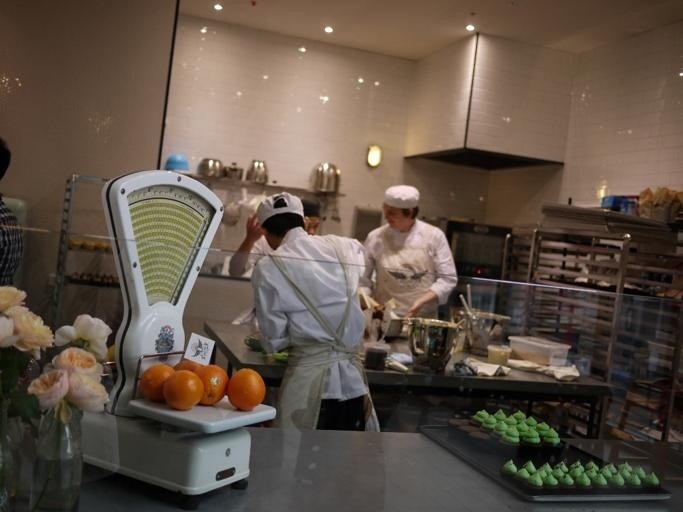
[486, 345, 512, 367]
[364, 343, 391, 371]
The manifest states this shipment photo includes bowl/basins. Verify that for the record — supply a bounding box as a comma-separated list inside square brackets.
[196, 158, 223, 177]
[408, 318, 458, 373]
[457, 310, 511, 354]
[311, 162, 341, 194]
[247, 159, 269, 183]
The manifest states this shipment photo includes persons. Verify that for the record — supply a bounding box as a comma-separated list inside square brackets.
[0, 140, 23, 286]
[251, 189, 379, 430]
[267, 431, 385, 511]
[222, 197, 325, 329]
[353, 183, 457, 326]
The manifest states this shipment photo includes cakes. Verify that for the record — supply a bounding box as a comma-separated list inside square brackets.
[447, 408, 567, 457]
[499, 459, 661, 495]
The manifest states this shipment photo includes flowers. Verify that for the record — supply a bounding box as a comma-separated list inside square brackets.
[0, 282, 114, 426]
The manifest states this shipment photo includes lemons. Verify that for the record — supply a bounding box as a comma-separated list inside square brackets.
[108, 344, 117, 370]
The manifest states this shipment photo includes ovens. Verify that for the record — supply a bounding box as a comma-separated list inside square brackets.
[438, 218, 515, 286]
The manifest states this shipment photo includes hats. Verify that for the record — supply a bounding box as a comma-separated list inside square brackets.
[384, 184, 419, 209]
[301, 198, 319, 218]
[256, 192, 304, 229]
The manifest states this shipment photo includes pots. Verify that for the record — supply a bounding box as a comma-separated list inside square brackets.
[224, 163, 244, 179]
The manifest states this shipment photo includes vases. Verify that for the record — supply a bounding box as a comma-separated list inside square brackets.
[0, 402, 84, 511]
